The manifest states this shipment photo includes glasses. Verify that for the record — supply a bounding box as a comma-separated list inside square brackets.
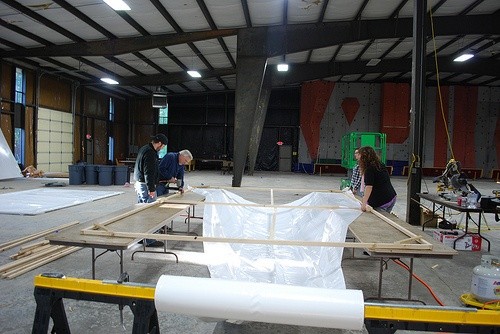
[184, 159, 188, 164]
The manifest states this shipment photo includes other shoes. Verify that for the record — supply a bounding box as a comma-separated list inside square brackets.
[162, 225, 171, 230]
[146, 241, 165, 247]
[138, 240, 143, 244]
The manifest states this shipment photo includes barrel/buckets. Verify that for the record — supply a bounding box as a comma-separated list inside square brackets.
[68, 164, 131, 185]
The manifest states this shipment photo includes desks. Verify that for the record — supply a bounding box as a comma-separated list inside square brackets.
[418, 192, 500, 252]
[315, 193, 460, 306]
[184, 159, 195, 172]
[45, 183, 212, 281]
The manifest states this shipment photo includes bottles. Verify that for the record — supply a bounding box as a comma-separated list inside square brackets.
[457, 191, 478, 209]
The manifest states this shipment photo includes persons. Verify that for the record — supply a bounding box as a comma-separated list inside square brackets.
[347, 146, 397, 212]
[133, 135, 193, 247]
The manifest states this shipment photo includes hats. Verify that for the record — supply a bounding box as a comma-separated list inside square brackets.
[153, 135, 165, 143]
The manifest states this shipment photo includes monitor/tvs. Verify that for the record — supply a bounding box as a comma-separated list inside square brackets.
[152, 94, 167, 108]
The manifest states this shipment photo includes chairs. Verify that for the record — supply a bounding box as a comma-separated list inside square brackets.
[221, 163, 230, 174]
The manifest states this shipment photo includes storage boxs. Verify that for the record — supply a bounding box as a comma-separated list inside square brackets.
[421, 211, 438, 227]
[434, 229, 481, 251]
[279, 145, 292, 172]
